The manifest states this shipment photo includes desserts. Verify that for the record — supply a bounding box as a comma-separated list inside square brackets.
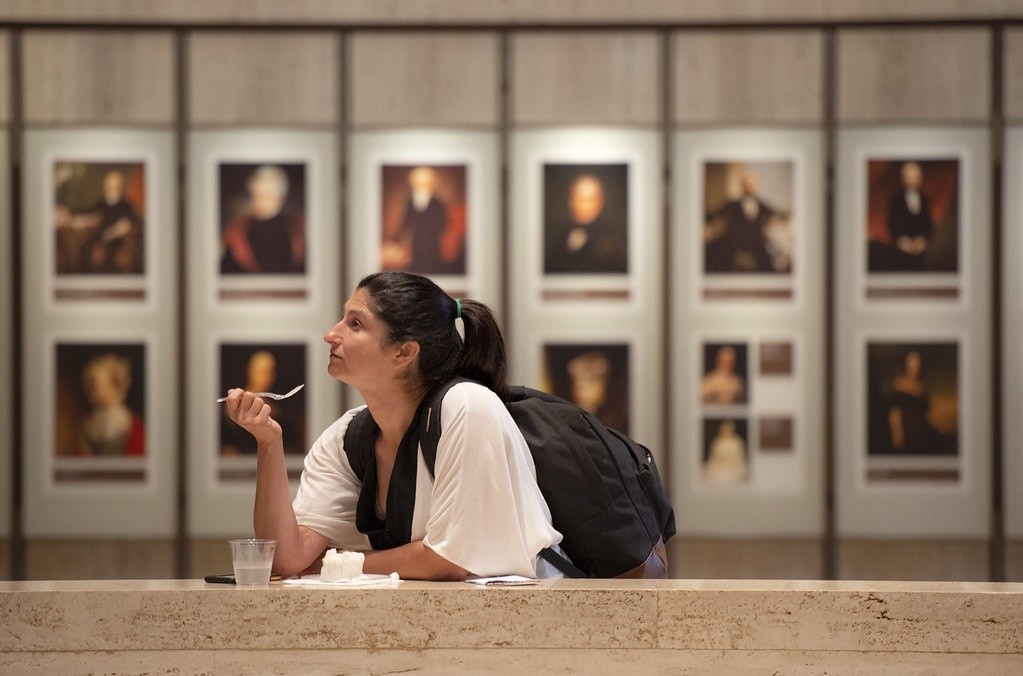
[320, 547, 365, 579]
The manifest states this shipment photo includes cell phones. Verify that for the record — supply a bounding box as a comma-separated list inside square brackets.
[205, 573, 282, 584]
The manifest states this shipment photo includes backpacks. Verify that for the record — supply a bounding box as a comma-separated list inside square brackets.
[342, 375, 676, 579]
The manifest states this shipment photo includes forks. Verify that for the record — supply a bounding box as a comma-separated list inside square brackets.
[216, 383, 304, 403]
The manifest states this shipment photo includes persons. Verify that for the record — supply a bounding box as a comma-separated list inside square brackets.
[65, 352, 143, 460]
[704, 419, 748, 477]
[883, 164, 935, 270]
[561, 349, 609, 418]
[222, 164, 294, 272]
[64, 171, 137, 273]
[393, 165, 452, 271]
[549, 175, 626, 269]
[224, 268, 575, 580]
[705, 344, 747, 402]
[882, 351, 948, 455]
[241, 349, 284, 452]
[709, 166, 789, 271]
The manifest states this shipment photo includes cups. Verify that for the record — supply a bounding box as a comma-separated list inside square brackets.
[228, 539, 277, 586]
